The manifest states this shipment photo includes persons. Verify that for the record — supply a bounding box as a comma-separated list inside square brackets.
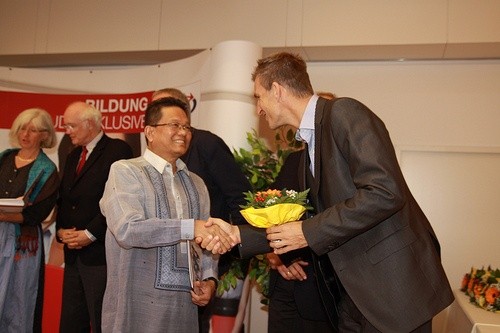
[195, 52, 454, 333]
[151, 87, 246, 333]
[55, 101, 134, 333]
[99, 98, 235, 333]
[0, 109, 60, 333]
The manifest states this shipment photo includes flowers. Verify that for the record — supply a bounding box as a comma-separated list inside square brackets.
[217, 128, 304, 296]
[460, 265, 500, 314]
[238, 188, 315, 252]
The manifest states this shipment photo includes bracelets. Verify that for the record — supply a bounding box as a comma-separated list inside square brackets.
[204, 277, 218, 286]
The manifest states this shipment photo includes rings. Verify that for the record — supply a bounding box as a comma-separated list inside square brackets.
[286, 271, 289, 275]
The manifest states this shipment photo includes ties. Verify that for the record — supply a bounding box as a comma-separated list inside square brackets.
[76, 145, 88, 175]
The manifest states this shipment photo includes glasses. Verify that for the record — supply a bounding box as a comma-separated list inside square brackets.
[150, 121, 191, 133]
[65, 124, 84, 130]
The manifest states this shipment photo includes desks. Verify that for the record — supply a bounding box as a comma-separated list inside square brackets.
[446, 290, 500, 333]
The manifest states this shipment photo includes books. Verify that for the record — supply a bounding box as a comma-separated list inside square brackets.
[0, 199, 25, 213]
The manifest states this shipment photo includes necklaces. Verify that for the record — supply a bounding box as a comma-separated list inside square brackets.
[17, 154, 33, 162]
